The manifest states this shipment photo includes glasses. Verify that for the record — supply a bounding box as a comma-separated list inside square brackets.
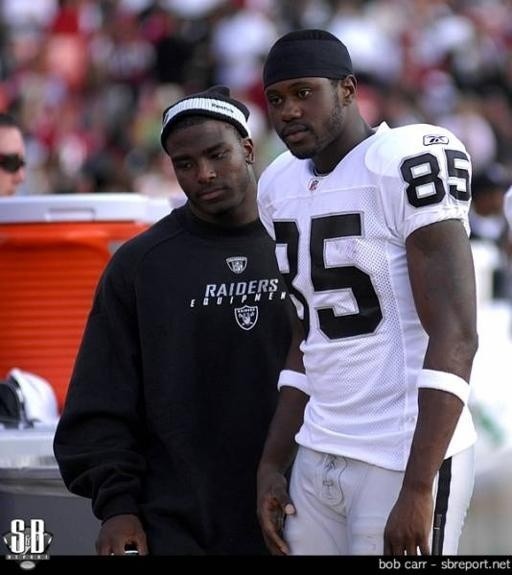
[0, 153, 26, 173]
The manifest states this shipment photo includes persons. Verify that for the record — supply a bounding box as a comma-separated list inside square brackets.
[256, 27, 479, 556]
[2, 1, 512, 303]
[52, 87, 294, 554]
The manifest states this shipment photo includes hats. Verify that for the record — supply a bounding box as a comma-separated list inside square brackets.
[160, 86, 250, 155]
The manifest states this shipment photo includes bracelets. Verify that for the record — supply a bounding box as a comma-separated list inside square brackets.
[414, 367, 471, 404]
[279, 371, 311, 399]
